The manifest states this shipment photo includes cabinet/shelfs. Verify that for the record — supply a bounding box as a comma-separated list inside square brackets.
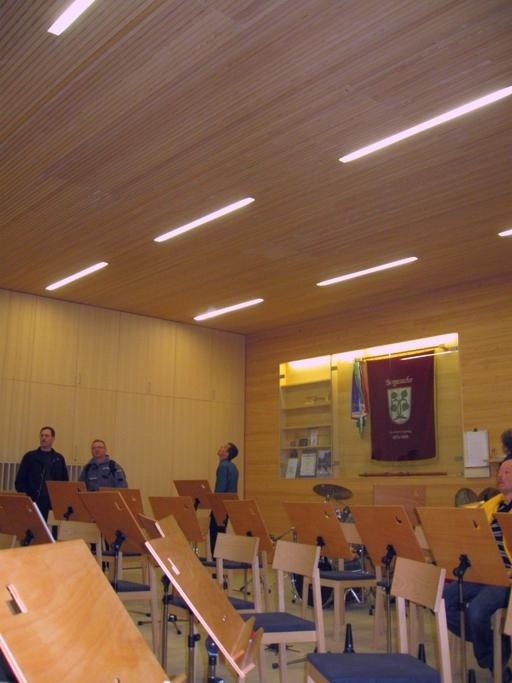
[279, 377, 339, 480]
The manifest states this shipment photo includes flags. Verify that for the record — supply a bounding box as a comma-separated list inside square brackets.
[349, 360, 365, 437]
[367, 350, 438, 466]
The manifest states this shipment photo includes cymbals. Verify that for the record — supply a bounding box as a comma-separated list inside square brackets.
[313, 484, 353, 500]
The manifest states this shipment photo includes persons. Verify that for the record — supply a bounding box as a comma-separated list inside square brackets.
[15, 426, 70, 544]
[499, 427, 512, 459]
[79, 437, 128, 572]
[209, 440, 240, 561]
[433, 453, 511, 682]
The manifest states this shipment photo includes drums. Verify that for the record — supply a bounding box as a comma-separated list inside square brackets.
[294, 546, 367, 610]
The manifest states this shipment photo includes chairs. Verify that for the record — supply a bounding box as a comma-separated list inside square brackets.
[168, 533, 262, 683]
[302, 521, 384, 642]
[303, 556, 452, 681]
[57, 521, 102, 573]
[238, 540, 326, 681]
[103, 556, 159, 659]
[451, 607, 508, 683]
[2, 521, 512, 681]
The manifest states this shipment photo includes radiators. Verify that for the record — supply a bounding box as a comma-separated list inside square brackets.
[0, 462, 86, 494]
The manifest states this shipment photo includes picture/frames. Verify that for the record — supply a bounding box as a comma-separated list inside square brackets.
[284, 449, 318, 480]
